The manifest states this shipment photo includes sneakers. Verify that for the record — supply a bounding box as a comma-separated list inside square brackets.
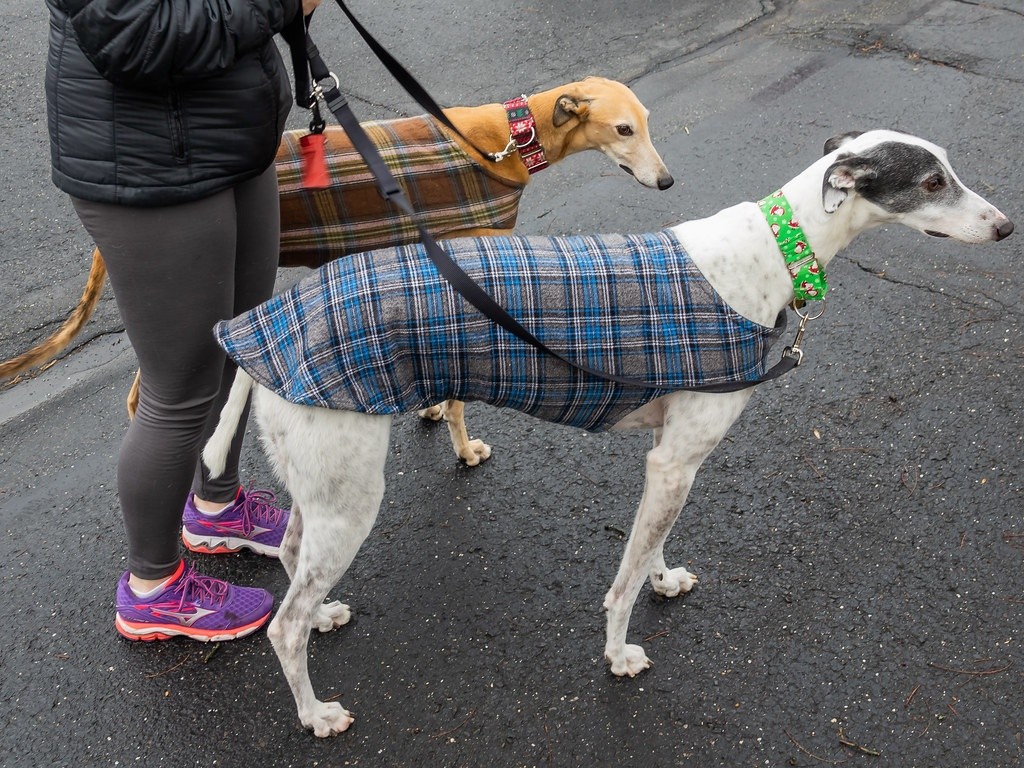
[114, 559, 274, 643]
[181, 479, 289, 559]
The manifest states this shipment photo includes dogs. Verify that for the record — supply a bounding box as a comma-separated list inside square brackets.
[196, 127, 1016, 739]
[0, 74, 677, 468]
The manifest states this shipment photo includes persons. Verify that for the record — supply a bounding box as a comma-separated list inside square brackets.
[45, 0, 325, 639]
